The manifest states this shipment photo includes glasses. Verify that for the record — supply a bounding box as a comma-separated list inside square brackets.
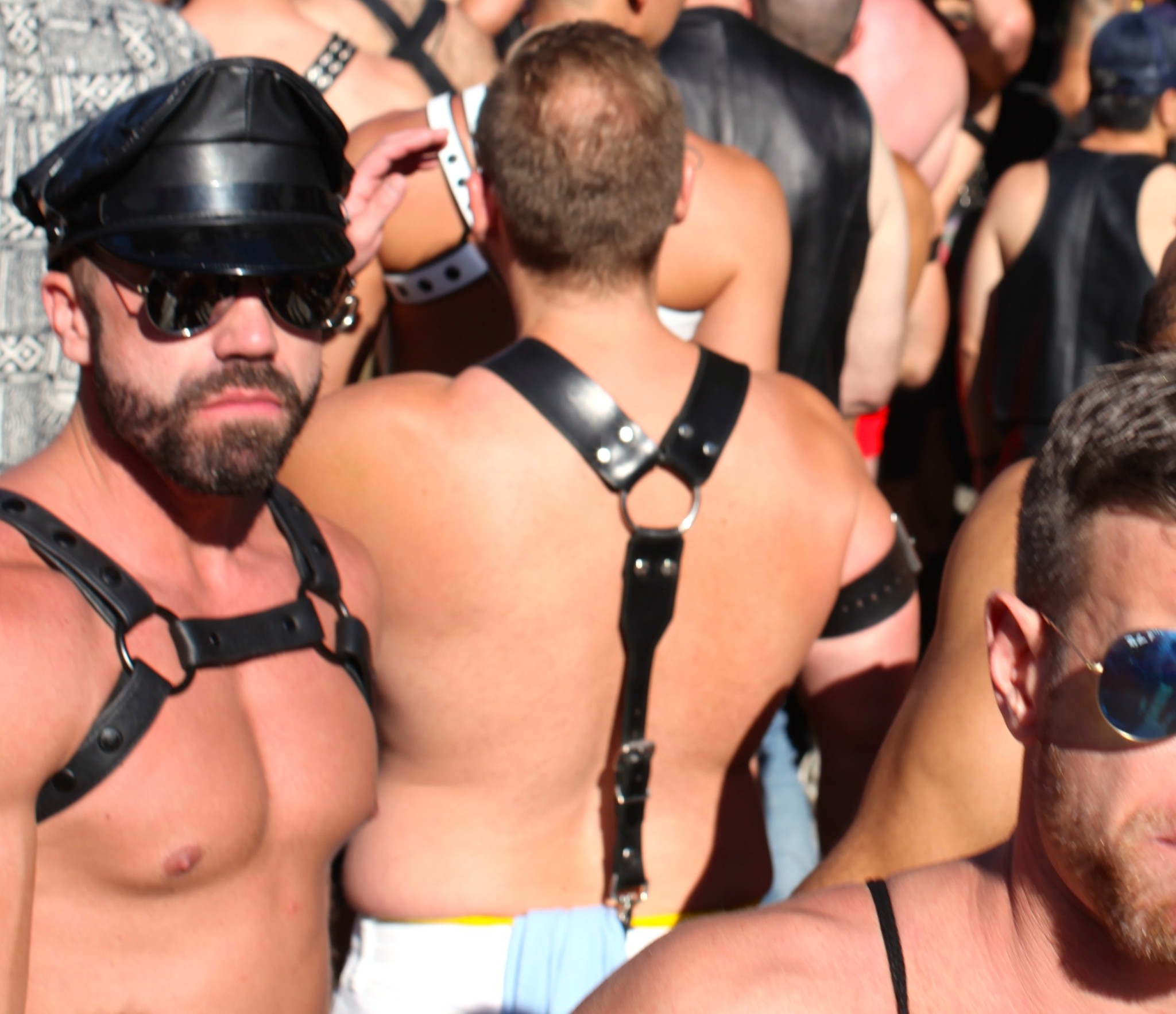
[80, 245, 350, 336]
[1029, 604, 1176, 743]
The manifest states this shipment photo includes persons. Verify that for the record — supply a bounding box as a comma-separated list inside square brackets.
[1, 0, 1176, 1014]
[273, 20, 924, 1014]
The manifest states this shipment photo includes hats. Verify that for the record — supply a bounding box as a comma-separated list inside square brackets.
[11, 58, 356, 277]
[1090, 14, 1176, 95]
